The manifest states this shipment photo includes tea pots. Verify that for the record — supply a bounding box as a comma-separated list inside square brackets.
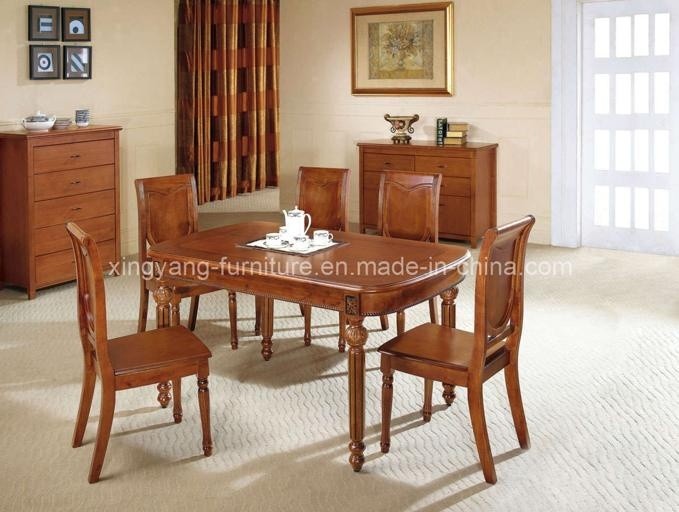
[282, 206, 312, 244]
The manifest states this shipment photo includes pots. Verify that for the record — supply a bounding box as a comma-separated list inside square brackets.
[22, 112, 56, 132]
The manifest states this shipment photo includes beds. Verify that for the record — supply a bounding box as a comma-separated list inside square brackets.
[143, 218, 476, 474]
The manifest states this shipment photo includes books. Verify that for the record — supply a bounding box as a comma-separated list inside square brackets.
[435, 116, 470, 147]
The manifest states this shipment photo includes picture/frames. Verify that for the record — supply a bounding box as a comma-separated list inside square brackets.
[62, 7, 94, 42]
[27, 5, 62, 41]
[348, 1, 455, 98]
[63, 45, 93, 80]
[27, 44, 63, 79]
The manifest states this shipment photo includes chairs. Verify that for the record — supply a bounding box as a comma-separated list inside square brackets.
[333, 168, 444, 358]
[132, 171, 244, 354]
[376, 210, 538, 486]
[251, 162, 355, 349]
[61, 217, 217, 486]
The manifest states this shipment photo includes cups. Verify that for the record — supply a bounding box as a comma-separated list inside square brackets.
[279, 226, 286, 240]
[314, 230, 334, 244]
[266, 232, 281, 247]
[75, 108, 90, 127]
[292, 236, 310, 248]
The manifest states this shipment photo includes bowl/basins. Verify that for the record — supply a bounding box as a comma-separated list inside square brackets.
[52, 118, 70, 129]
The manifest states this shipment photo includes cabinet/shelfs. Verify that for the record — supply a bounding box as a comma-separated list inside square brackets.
[354, 138, 501, 250]
[0, 125, 126, 302]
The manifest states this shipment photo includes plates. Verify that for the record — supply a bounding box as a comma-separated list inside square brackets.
[311, 241, 331, 246]
[291, 248, 309, 252]
[264, 242, 288, 248]
